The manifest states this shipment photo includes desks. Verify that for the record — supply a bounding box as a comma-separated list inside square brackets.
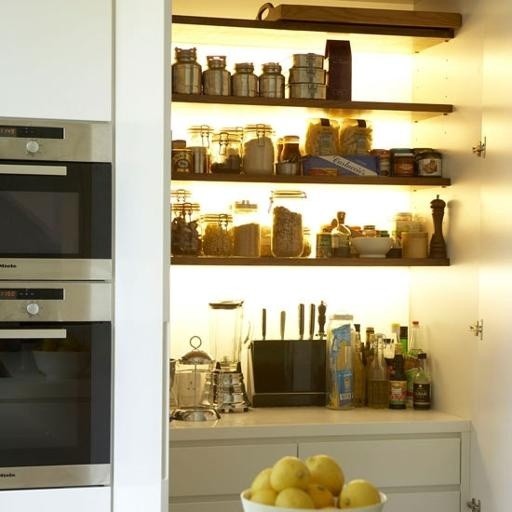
[169, 405, 472, 512]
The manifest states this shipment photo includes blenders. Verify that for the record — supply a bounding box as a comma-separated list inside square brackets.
[209, 298, 251, 411]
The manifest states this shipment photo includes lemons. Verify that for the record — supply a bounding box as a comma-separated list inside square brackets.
[249, 453, 379, 510]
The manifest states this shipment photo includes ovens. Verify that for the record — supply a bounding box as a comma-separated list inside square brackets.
[0, 112, 113, 491]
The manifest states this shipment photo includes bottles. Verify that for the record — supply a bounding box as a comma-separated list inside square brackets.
[354, 323, 364, 354]
[366, 336, 389, 408]
[404, 321, 424, 408]
[331, 211, 351, 257]
[389, 342, 408, 409]
[383, 321, 401, 369]
[348, 331, 365, 408]
[365, 327, 374, 361]
[414, 353, 431, 409]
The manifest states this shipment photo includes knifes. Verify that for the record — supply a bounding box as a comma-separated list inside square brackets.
[261, 307, 267, 340]
[315, 300, 327, 339]
[280, 311, 286, 340]
[297, 303, 304, 340]
[309, 303, 315, 340]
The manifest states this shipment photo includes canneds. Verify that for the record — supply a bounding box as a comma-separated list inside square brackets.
[413, 150, 443, 178]
[171, 148, 194, 173]
[394, 152, 415, 177]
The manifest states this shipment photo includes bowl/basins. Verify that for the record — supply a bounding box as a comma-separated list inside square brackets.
[349, 237, 394, 258]
[240, 488, 387, 512]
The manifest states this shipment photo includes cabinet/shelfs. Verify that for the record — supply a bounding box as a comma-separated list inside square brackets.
[170, 15, 456, 266]
